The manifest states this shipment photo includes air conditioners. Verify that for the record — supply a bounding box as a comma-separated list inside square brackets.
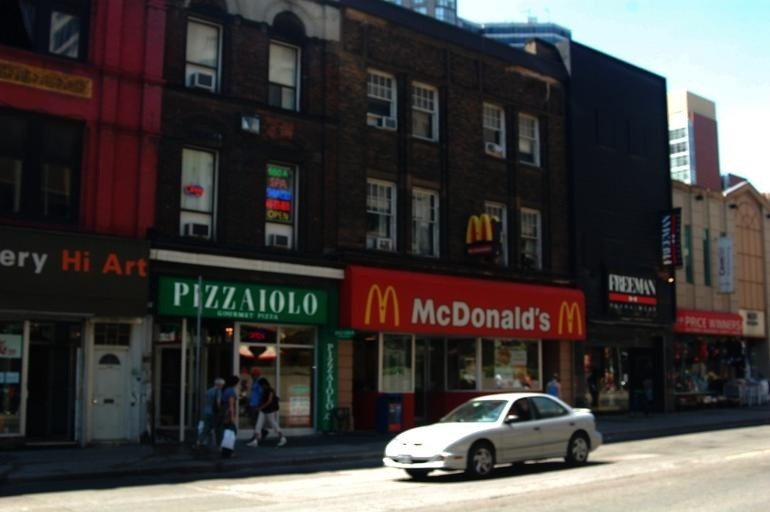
[186, 222, 212, 240]
[273, 234, 289, 249]
[375, 238, 393, 252]
[378, 116, 397, 131]
[191, 71, 216, 92]
[485, 141, 501, 157]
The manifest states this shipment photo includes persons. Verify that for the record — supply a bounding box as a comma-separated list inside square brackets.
[244, 377, 288, 447]
[190, 377, 226, 458]
[584, 364, 601, 407]
[217, 374, 239, 457]
[546, 372, 562, 401]
[247, 366, 270, 442]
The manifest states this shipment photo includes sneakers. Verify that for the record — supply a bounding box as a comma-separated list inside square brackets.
[246, 429, 287, 448]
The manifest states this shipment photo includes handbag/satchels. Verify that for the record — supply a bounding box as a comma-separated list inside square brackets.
[221, 428, 235, 451]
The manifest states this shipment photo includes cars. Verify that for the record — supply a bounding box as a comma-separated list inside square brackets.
[383, 391, 601, 478]
